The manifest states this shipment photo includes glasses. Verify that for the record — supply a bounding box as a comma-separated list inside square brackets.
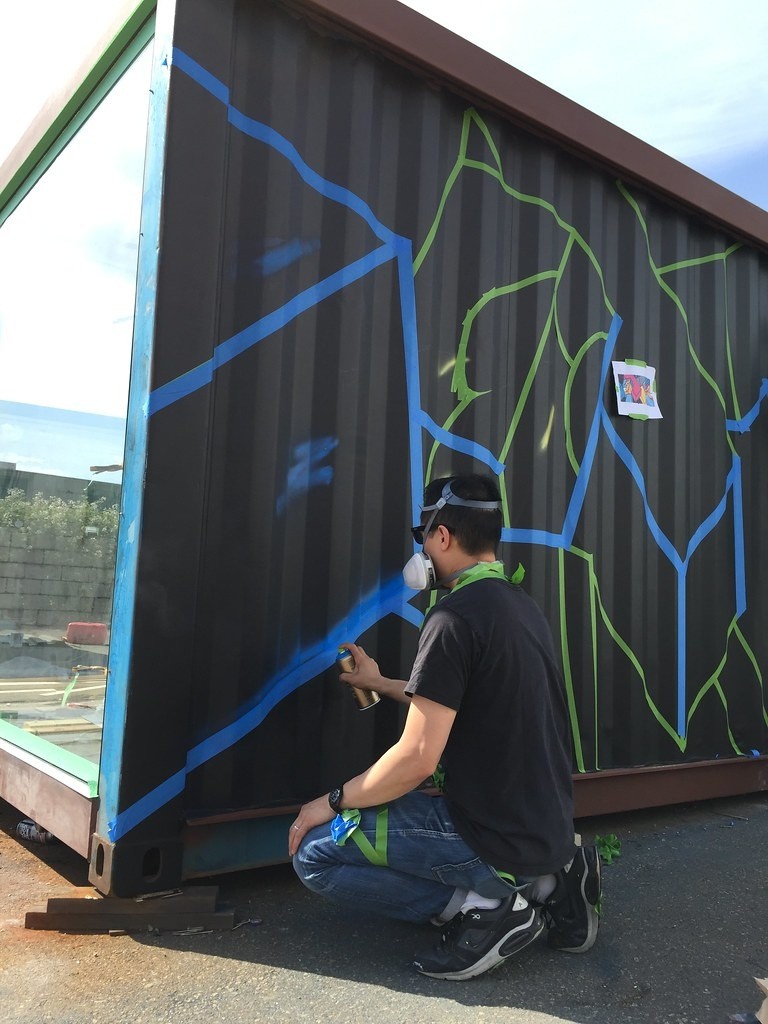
[410, 524, 455, 544]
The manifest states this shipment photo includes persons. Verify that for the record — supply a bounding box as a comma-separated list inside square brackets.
[287, 473, 603, 984]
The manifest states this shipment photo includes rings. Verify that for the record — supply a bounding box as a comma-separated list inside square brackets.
[294, 826, 299, 831]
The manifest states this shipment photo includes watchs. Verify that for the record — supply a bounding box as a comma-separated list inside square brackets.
[328, 783, 345, 815]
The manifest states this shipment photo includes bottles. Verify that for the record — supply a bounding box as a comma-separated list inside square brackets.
[336, 648, 381, 711]
[16, 819, 54, 844]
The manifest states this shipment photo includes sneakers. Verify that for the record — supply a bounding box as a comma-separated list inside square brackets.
[544, 845, 602, 955]
[410, 889, 545, 981]
